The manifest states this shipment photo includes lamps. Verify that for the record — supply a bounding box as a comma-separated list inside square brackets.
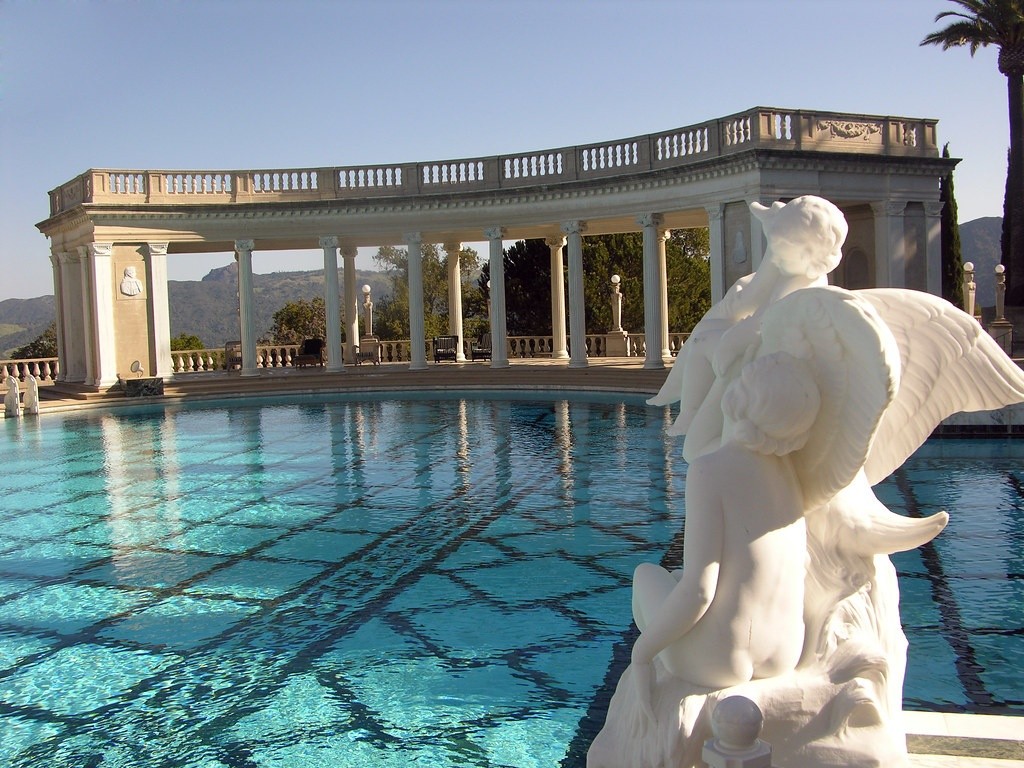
[130, 360, 144, 378]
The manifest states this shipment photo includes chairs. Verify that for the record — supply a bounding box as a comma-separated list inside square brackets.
[225, 332, 492, 373]
